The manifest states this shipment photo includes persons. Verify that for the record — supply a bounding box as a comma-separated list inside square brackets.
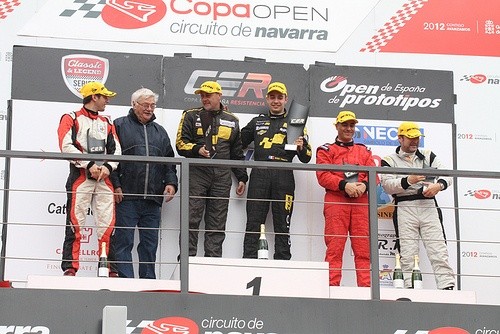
[58, 81, 122, 277]
[381, 122, 456, 289]
[176, 81, 248, 261]
[109, 87, 178, 278]
[240, 82, 312, 259]
[316, 111, 380, 287]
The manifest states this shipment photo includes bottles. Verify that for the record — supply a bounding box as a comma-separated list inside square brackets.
[97, 242, 109, 277]
[393, 254, 403, 288]
[258, 224, 268, 259]
[412, 255, 422, 289]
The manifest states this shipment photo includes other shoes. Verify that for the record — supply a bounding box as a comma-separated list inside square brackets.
[64, 271, 75, 276]
[109, 272, 118, 277]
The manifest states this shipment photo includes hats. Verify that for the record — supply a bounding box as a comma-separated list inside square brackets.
[195, 81, 222, 94]
[398, 122, 425, 139]
[267, 82, 287, 98]
[80, 81, 117, 99]
[335, 111, 358, 125]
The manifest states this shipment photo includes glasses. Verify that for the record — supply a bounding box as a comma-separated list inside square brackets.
[137, 102, 157, 109]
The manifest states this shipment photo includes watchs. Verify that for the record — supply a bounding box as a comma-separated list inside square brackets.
[441, 184, 444, 189]
